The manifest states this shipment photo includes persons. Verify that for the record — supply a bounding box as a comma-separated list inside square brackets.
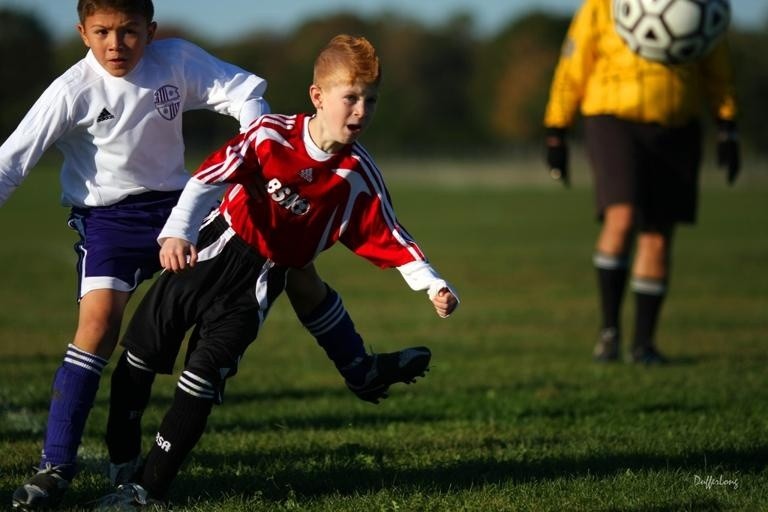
[1, 1, 431, 512]
[544, 1, 745, 369]
[93, 28, 460, 512]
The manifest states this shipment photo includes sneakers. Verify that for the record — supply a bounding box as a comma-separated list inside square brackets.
[85, 458, 160, 512]
[592, 329, 669, 380]
[344, 343, 433, 408]
[9, 459, 82, 511]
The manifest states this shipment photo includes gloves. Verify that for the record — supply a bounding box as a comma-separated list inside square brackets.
[714, 117, 744, 184]
[543, 140, 572, 191]
